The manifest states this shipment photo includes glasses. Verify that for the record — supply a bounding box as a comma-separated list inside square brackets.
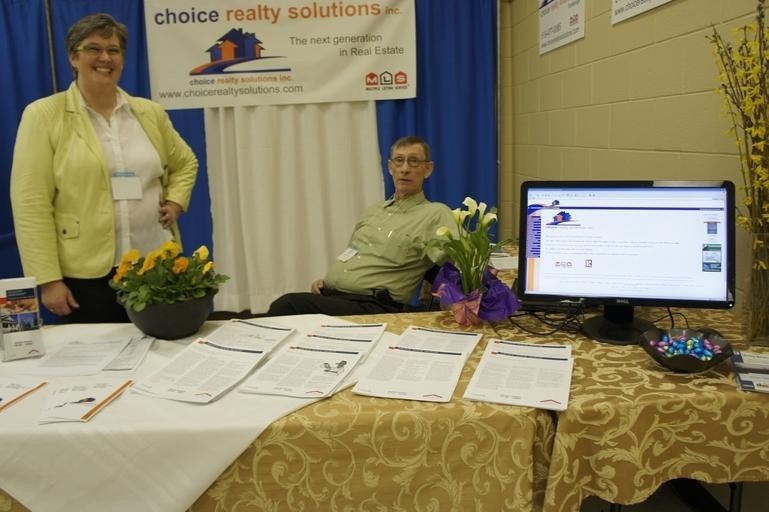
[390, 156, 427, 167]
[75, 47, 121, 55]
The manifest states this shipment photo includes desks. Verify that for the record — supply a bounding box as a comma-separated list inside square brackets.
[487, 241, 520, 289]
[0, 310, 554, 512]
[493, 304, 769, 511]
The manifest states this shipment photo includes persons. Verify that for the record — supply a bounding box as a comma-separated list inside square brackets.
[265, 135, 458, 317]
[9, 13, 200, 323]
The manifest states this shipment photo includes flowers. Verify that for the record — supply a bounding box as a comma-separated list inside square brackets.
[110, 240, 231, 312]
[420, 195, 522, 294]
[707, 1, 769, 346]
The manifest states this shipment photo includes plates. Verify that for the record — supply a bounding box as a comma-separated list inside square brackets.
[638, 328, 734, 372]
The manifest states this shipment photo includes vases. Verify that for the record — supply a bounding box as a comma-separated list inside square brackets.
[118, 288, 218, 339]
[450, 294, 482, 325]
[745, 231, 769, 346]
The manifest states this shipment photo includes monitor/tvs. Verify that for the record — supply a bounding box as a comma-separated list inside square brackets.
[516, 180, 737, 345]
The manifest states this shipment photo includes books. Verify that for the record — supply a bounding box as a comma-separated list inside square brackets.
[129, 318, 297, 404]
[351, 324, 484, 402]
[0, 275, 48, 363]
[732, 348, 769, 394]
[0, 376, 49, 411]
[239, 323, 386, 400]
[37, 377, 134, 426]
[460, 337, 574, 411]
